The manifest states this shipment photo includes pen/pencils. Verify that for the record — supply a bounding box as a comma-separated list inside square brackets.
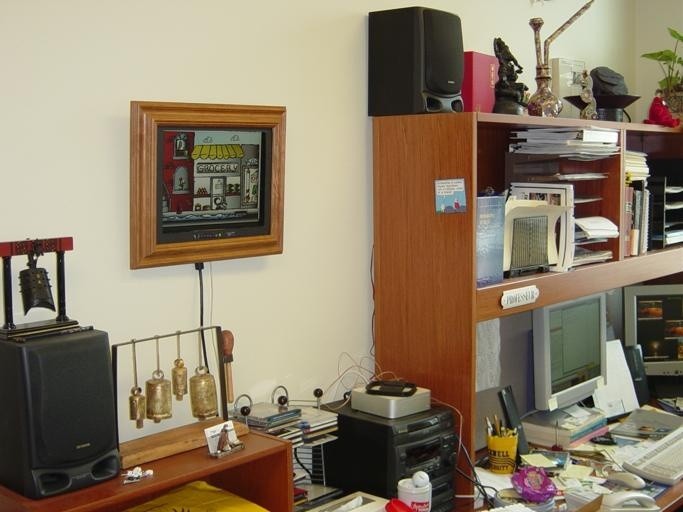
[484, 414, 519, 437]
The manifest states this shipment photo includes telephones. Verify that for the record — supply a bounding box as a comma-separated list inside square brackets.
[603, 491, 661, 511]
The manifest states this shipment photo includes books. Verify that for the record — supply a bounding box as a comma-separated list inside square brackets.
[227, 399, 338, 507]
[505, 125, 683, 273]
[521, 399, 683, 449]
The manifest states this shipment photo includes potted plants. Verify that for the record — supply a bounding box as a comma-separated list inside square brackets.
[638, 26, 683, 119]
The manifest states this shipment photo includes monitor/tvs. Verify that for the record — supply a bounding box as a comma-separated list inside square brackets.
[531, 290, 608, 420]
[622, 285, 683, 376]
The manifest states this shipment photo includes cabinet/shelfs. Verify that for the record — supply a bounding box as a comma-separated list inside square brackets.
[368, 108, 683, 512]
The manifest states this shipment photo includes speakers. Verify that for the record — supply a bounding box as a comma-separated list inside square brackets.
[498, 383, 531, 458]
[624, 344, 652, 405]
[369, 6, 465, 115]
[0, 324, 121, 498]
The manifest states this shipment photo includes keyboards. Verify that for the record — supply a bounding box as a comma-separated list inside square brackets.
[618, 422, 683, 487]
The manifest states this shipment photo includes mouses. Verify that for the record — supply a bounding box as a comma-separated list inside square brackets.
[606, 469, 646, 490]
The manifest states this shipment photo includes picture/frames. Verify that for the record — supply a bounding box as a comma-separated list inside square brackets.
[129, 98, 287, 268]
